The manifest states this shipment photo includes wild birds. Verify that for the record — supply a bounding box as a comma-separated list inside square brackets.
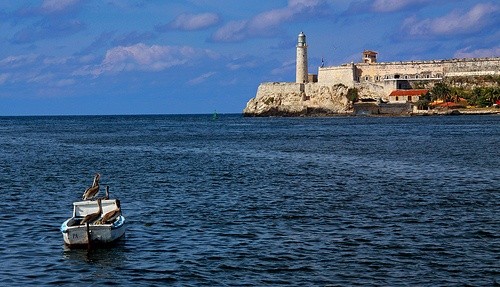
[99, 199, 121, 224]
[96, 185, 110, 200]
[81, 198, 104, 225]
[81, 173, 100, 201]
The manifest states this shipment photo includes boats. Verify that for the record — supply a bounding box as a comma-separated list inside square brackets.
[60, 199, 125, 246]
